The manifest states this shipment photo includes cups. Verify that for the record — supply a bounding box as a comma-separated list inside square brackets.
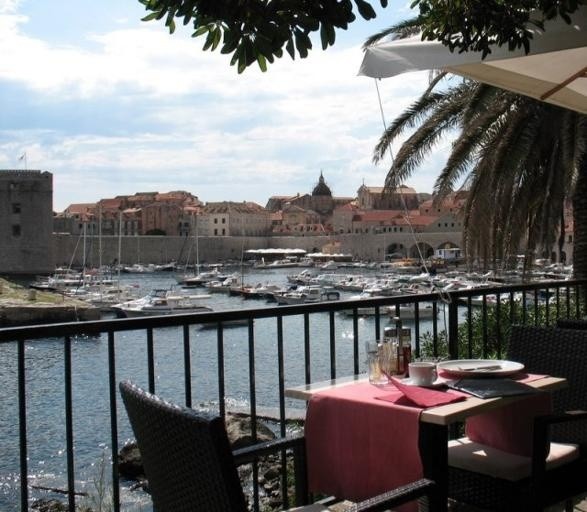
[408, 361, 437, 387]
[365, 339, 392, 385]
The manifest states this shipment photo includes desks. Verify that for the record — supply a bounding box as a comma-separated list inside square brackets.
[283, 360, 570, 512]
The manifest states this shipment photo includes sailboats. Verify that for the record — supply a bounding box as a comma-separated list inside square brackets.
[30, 202, 248, 309]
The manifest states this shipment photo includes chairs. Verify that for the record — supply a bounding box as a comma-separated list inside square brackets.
[448, 323, 587, 511]
[119, 378, 441, 512]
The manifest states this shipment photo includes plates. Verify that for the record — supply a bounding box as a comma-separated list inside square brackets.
[438, 359, 526, 379]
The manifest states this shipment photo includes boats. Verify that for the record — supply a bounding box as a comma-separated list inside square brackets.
[250, 248, 575, 319]
[111, 290, 213, 318]
